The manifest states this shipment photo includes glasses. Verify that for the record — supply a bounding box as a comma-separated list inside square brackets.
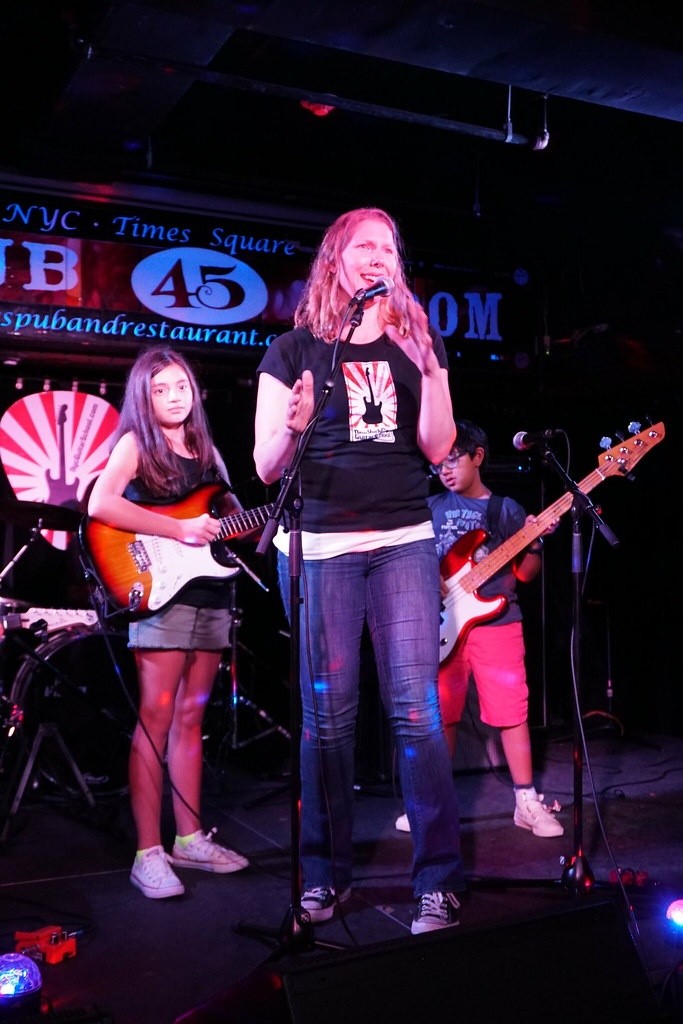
[429, 447, 469, 475]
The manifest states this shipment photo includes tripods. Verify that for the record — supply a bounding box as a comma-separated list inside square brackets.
[232, 297, 356, 978]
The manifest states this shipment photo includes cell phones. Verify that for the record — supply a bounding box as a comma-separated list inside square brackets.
[462, 444, 666, 905]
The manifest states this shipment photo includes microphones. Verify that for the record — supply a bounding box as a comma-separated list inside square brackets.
[513, 430, 563, 448]
[347, 276, 395, 308]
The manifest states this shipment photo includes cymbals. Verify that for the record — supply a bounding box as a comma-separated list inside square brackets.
[0, 499, 88, 533]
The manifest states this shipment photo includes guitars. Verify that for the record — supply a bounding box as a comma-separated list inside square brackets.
[439, 418, 666, 671]
[79, 484, 284, 619]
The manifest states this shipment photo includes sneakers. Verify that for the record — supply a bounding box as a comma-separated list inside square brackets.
[512, 797, 565, 837]
[130, 843, 185, 898]
[394, 812, 414, 832]
[410, 889, 460, 935]
[171, 827, 251, 872]
[301, 883, 351, 923]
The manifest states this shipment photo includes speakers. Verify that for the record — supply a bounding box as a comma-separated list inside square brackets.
[278, 892, 665, 1024]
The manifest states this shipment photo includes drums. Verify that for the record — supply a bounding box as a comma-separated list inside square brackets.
[11, 622, 140, 791]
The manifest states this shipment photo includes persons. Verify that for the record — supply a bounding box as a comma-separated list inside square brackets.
[395, 423, 565, 839]
[87, 347, 263, 900]
[253, 206, 467, 936]
